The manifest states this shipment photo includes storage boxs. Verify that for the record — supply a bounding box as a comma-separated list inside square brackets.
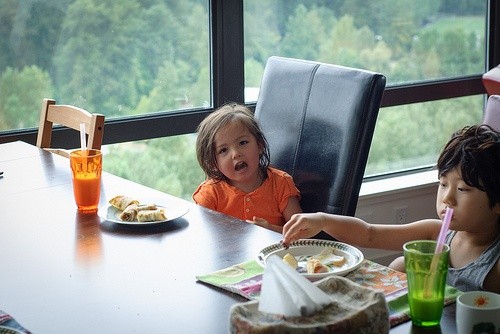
[228, 274, 390, 333]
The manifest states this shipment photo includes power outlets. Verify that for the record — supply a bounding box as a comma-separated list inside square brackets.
[394, 205, 410, 224]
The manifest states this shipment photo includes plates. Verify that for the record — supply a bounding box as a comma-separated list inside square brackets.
[97, 199, 190, 225]
[254, 239, 364, 282]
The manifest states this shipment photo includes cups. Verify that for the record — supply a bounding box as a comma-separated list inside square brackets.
[456, 291, 500, 334]
[69, 149, 103, 215]
[403, 240, 450, 327]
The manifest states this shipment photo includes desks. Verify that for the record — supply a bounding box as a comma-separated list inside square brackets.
[0, 140, 499, 333]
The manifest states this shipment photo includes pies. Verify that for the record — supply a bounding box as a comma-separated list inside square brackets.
[109, 194, 167, 222]
[282, 247, 346, 274]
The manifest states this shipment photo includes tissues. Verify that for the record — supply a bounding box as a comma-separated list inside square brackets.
[228, 255, 391, 334]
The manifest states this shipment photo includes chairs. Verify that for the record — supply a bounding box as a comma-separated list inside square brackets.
[251, 54, 387, 241]
[35, 98, 106, 162]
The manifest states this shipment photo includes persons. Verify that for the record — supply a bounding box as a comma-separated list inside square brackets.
[278, 123, 500, 296]
[192, 102, 303, 235]
